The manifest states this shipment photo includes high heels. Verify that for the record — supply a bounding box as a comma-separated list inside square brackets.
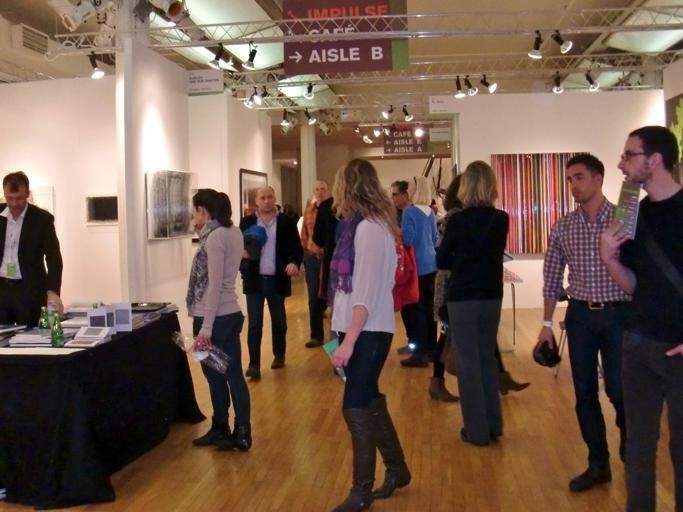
[500, 371, 531, 395]
[429, 376, 460, 402]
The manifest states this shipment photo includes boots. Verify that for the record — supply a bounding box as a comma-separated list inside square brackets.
[368, 393, 411, 499]
[332, 409, 381, 511]
[216, 417, 252, 451]
[192, 414, 231, 447]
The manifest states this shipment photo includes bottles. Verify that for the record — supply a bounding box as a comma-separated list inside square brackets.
[38, 306, 50, 330]
[50, 313, 63, 348]
[92, 303, 97, 308]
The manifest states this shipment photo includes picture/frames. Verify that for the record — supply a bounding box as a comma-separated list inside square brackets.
[239, 168, 269, 224]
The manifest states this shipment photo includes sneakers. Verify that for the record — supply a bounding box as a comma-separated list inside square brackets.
[246, 366, 260, 378]
[305, 339, 324, 347]
[397, 346, 412, 354]
[271, 355, 285, 368]
[568, 465, 612, 492]
[400, 356, 429, 368]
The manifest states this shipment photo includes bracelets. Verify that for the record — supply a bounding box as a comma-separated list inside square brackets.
[542, 321, 554, 328]
[603, 255, 615, 266]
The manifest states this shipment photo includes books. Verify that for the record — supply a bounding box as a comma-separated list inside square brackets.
[0, 301, 179, 349]
[612, 180, 642, 241]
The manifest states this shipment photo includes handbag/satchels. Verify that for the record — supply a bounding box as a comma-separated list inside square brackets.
[391, 238, 420, 312]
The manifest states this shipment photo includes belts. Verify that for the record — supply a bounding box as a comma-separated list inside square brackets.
[576, 301, 617, 310]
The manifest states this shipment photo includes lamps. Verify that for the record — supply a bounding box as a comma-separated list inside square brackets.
[583, 70, 600, 93]
[552, 72, 564, 95]
[204, 42, 498, 142]
[86, 52, 106, 82]
[550, 27, 574, 54]
[526, 29, 544, 61]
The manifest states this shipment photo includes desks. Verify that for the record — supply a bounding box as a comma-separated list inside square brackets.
[0, 301, 200, 511]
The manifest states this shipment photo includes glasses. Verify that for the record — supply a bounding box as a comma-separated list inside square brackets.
[621, 149, 645, 161]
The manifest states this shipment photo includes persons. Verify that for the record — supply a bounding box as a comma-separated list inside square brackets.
[538, 153, 625, 492]
[0, 170, 65, 332]
[598, 125, 682, 512]
[185, 187, 252, 452]
[387, 160, 533, 447]
[239, 179, 342, 381]
[330, 160, 411, 512]
